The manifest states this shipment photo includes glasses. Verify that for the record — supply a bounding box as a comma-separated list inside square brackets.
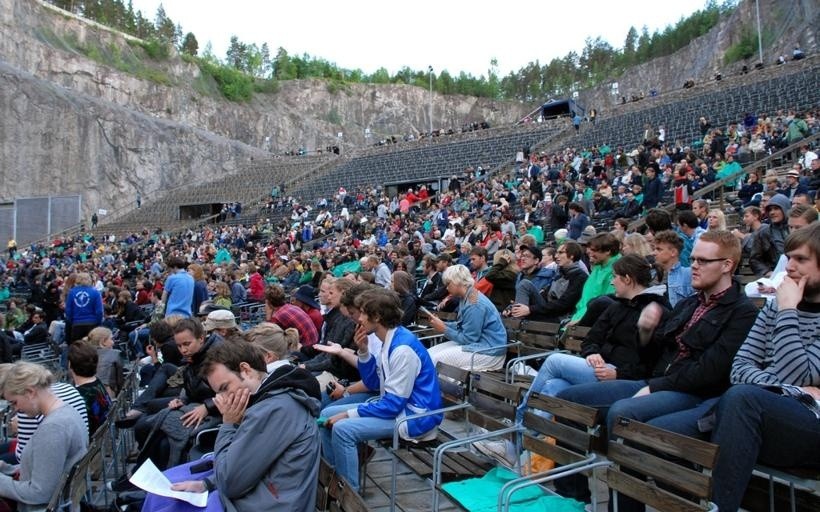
[555, 250, 569, 255]
[688, 256, 726, 265]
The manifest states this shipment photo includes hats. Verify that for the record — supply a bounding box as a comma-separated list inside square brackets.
[741, 169, 801, 209]
[520, 245, 542, 262]
[201, 309, 235, 331]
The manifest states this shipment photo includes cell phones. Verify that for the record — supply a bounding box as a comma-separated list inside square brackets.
[757, 282, 763, 286]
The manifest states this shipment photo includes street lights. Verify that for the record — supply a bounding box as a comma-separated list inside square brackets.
[428, 65, 435, 137]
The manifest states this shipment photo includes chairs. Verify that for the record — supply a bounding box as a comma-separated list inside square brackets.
[36, 146, 365, 251]
[1, 287, 819, 510]
[366, 58, 820, 249]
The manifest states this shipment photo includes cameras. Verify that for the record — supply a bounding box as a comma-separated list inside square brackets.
[326, 378, 349, 395]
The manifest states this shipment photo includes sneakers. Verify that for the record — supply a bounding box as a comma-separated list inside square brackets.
[114, 413, 143, 430]
[106, 472, 136, 491]
[468, 435, 517, 469]
